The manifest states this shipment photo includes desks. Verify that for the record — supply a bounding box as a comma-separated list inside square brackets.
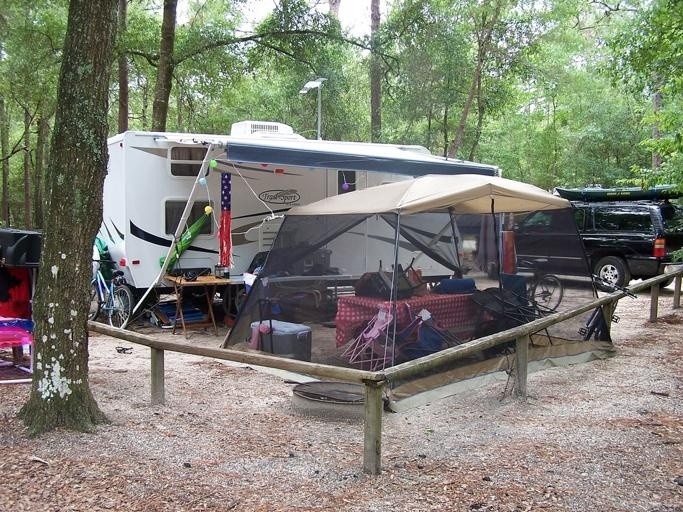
[164, 273, 229, 340]
[257, 276, 363, 316]
[337, 290, 472, 343]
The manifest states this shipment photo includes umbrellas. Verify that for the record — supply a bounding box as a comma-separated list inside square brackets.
[118, 206, 212, 328]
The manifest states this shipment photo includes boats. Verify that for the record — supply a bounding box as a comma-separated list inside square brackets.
[555, 184, 679, 200]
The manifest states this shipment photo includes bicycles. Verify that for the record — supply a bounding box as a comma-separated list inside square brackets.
[90, 259, 133, 329]
[578, 273, 637, 341]
[519, 258, 563, 313]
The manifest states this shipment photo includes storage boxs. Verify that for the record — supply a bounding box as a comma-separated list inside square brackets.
[252, 316, 314, 357]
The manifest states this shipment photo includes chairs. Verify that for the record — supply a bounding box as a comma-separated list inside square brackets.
[471, 270, 560, 347]
[342, 309, 399, 370]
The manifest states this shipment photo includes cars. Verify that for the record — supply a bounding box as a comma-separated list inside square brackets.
[511, 199, 683, 292]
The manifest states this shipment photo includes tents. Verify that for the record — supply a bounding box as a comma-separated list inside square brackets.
[216, 173, 622, 410]
